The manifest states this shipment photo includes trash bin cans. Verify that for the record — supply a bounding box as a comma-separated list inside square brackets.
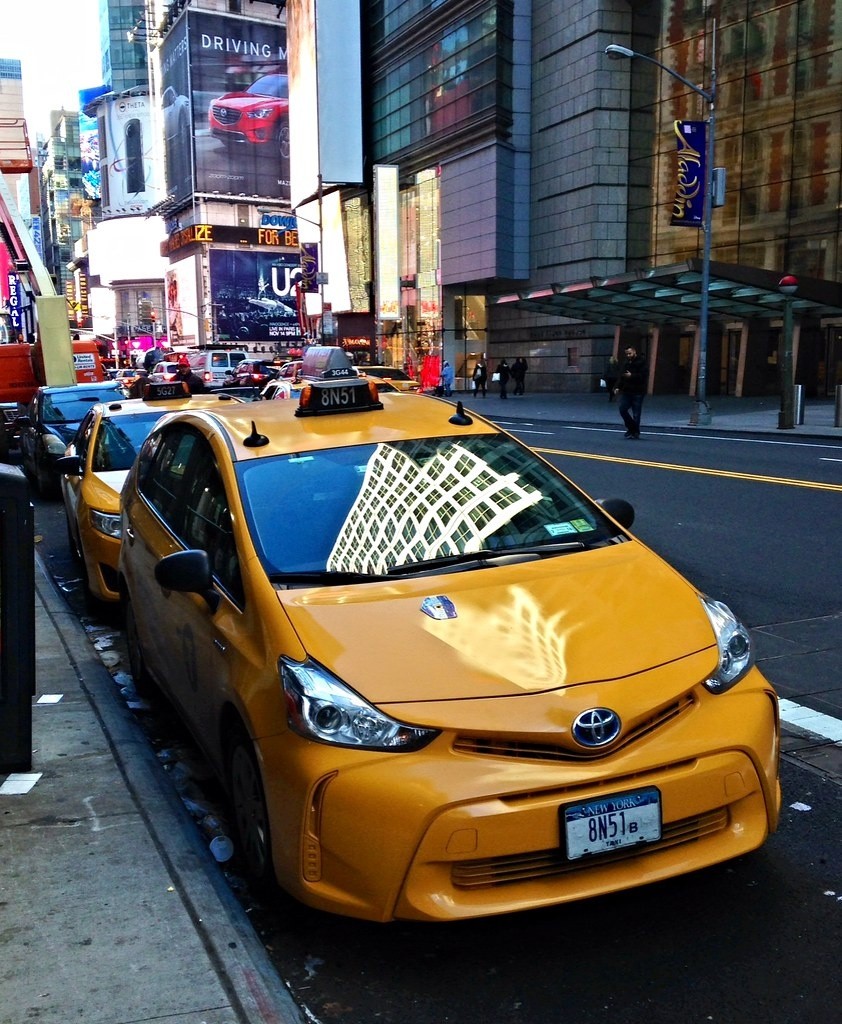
[834, 384, 842, 427]
[0, 460, 34, 775]
[795, 384, 806, 425]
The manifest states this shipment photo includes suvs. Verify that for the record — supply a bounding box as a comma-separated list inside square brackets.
[209, 73, 290, 159]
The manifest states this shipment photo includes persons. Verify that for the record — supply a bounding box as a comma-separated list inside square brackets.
[513, 357, 528, 395]
[377, 362, 392, 377]
[170, 358, 204, 395]
[146, 346, 175, 374]
[440, 361, 453, 396]
[472, 360, 487, 397]
[605, 345, 649, 439]
[493, 359, 512, 399]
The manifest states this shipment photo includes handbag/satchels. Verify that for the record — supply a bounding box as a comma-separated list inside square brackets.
[474, 368, 481, 380]
[491, 373, 500, 381]
[472, 381, 476, 390]
[600, 378, 607, 387]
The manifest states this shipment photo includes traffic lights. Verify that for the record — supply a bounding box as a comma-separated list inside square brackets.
[150, 310, 156, 322]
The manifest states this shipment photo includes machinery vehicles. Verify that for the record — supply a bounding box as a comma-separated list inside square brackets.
[0, 159, 104, 460]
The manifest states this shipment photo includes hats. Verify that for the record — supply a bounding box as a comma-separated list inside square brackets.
[178, 357, 190, 367]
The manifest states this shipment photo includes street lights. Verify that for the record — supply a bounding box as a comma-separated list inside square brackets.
[604, 43, 719, 424]
[257, 207, 325, 346]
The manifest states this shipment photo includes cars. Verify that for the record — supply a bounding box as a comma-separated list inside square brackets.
[119, 381, 781, 922]
[147, 362, 179, 381]
[260, 379, 314, 399]
[53, 381, 247, 601]
[352, 366, 423, 394]
[15, 387, 126, 492]
[105, 368, 147, 388]
[274, 361, 303, 380]
[225, 359, 286, 397]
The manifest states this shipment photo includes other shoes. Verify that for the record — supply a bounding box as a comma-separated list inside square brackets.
[624, 431, 639, 439]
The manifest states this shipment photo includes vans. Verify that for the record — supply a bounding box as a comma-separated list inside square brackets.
[189, 350, 248, 388]
